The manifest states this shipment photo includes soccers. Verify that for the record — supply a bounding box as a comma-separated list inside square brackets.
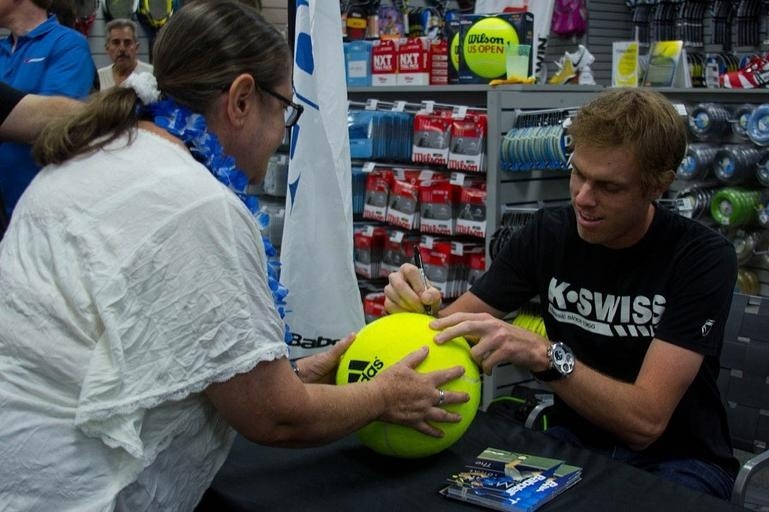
[337, 312, 481, 457]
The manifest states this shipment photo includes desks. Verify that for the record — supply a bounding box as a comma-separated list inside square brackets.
[208, 412, 737, 511]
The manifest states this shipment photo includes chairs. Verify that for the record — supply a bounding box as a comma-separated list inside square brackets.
[717, 287, 767, 508]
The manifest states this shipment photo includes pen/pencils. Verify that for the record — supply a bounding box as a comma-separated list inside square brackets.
[414, 245, 432, 315]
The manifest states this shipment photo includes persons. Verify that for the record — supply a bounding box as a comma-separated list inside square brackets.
[384, 89, 740, 500]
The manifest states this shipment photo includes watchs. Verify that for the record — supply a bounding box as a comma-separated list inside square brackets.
[530, 341, 575, 382]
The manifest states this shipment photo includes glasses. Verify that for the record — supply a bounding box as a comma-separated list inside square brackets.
[256, 80, 303, 128]
